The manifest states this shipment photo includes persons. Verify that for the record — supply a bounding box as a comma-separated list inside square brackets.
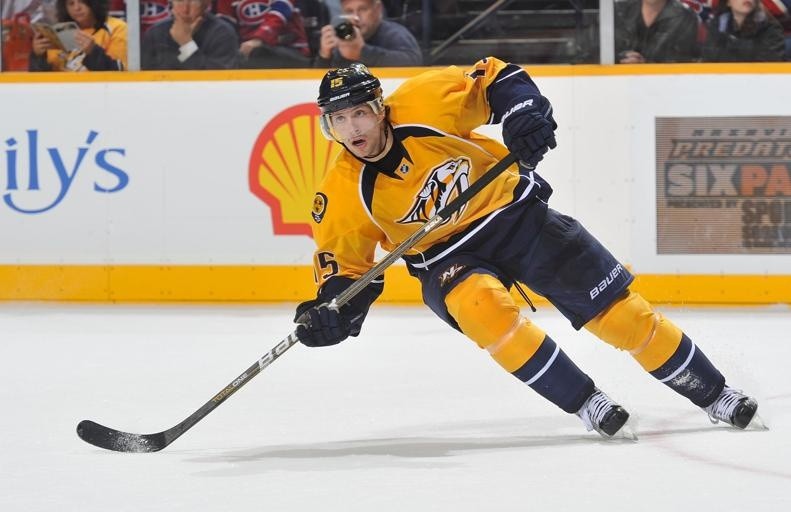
[292, 51, 759, 434]
[579, 0, 791, 64]
[28, 0, 311, 71]
[316, 0, 423, 68]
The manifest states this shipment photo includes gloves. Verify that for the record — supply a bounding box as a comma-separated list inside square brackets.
[295, 275, 373, 348]
[501, 95, 557, 167]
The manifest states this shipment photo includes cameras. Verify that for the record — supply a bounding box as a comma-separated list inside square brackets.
[332, 17, 354, 39]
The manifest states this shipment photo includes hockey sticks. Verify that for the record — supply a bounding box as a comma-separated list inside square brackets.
[76, 152, 518, 451]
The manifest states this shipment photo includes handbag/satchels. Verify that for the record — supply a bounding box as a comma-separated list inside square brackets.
[1, 13, 33, 70]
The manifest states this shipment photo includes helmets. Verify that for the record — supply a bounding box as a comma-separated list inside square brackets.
[318, 62, 388, 143]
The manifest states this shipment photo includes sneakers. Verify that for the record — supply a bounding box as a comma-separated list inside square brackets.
[701, 383, 758, 427]
[572, 387, 628, 437]
